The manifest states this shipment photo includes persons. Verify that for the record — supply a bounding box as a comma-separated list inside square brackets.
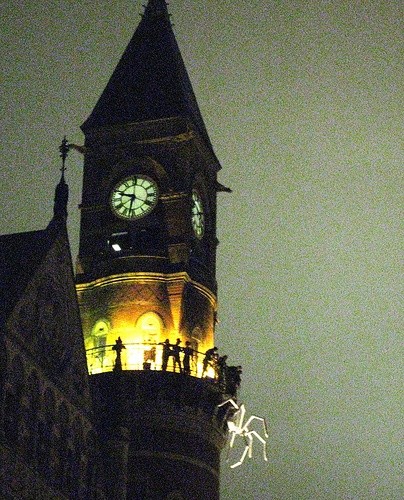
[172, 338, 183, 373]
[158, 339, 173, 371]
[202, 347, 218, 379]
[230, 365, 243, 376]
[183, 341, 194, 374]
[218, 354, 228, 364]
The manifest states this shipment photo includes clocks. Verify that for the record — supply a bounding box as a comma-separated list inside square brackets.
[192, 188, 206, 241]
[109, 174, 159, 219]
